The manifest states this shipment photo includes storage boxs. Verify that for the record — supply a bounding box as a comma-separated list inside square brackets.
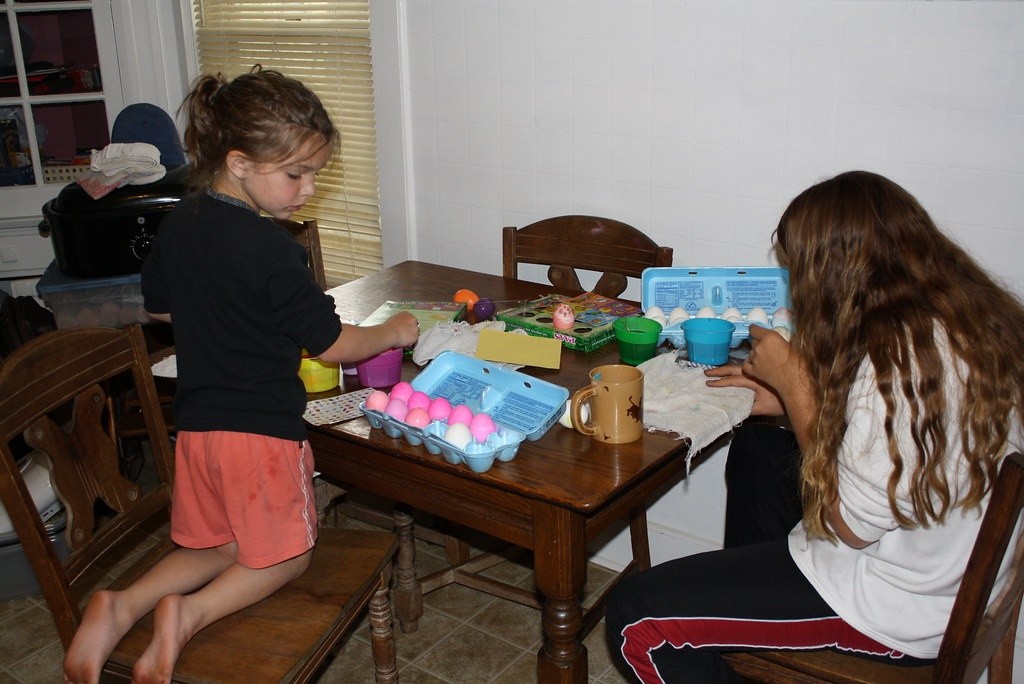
[497, 294, 619, 351]
[37, 258, 154, 331]
[359, 299, 469, 354]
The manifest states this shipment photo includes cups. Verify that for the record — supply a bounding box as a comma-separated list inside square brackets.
[297, 356, 340, 394]
[570, 364, 644, 444]
[355, 347, 404, 388]
[681, 317, 736, 366]
[612, 316, 664, 366]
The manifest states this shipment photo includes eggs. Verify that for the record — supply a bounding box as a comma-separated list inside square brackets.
[454, 289, 496, 319]
[646, 305, 791, 343]
[559, 399, 589, 429]
[553, 305, 576, 328]
[365, 382, 496, 450]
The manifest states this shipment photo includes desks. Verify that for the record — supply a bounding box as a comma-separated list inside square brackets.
[161, 259, 773, 683]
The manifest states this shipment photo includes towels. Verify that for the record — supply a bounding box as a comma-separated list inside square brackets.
[411, 318, 530, 371]
[76, 142, 167, 200]
[575, 348, 755, 458]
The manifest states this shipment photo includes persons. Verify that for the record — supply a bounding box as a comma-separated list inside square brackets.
[604, 169, 1024, 684]
[65, 62, 422, 684]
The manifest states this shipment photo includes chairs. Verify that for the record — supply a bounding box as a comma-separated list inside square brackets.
[0, 325, 399, 684]
[334, 216, 672, 654]
[724, 449, 1024, 684]
